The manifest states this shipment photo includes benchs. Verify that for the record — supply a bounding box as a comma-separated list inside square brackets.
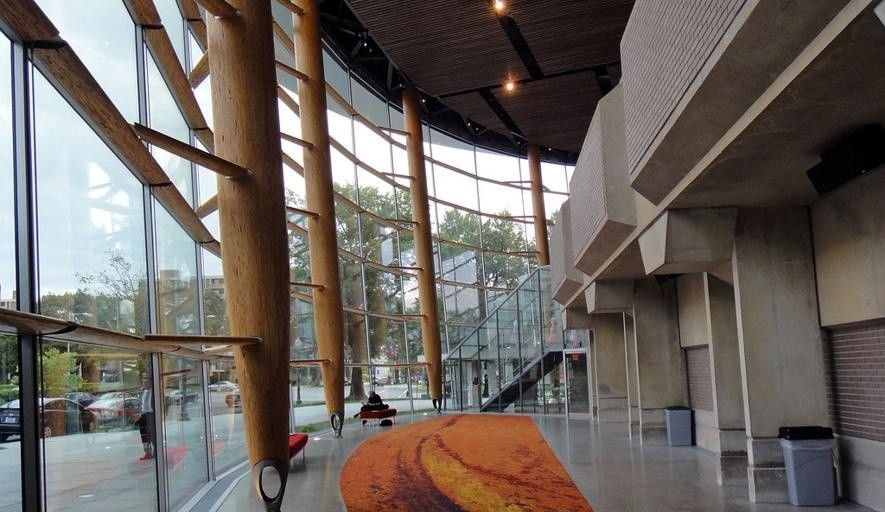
[358, 407, 397, 428]
[285, 431, 309, 464]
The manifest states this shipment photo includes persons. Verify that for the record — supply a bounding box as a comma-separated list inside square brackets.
[362, 391, 388, 412]
[137, 371, 156, 461]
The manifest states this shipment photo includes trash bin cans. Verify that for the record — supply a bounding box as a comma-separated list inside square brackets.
[664, 407, 692, 447]
[778, 427, 835, 507]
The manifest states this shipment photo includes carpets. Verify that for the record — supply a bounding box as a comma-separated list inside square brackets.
[339, 414, 597, 511]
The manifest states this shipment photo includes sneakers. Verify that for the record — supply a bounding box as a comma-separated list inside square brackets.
[140, 454, 152, 460]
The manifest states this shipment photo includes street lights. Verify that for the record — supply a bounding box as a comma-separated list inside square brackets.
[294, 336, 303, 405]
[176, 312, 217, 336]
[67, 311, 93, 375]
[177, 313, 190, 421]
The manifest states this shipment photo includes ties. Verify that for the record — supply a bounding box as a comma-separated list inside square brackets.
[144, 391, 149, 411]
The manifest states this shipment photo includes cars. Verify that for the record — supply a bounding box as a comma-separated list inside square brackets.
[99, 392, 133, 398]
[208, 380, 237, 391]
[225, 389, 241, 408]
[440, 376, 452, 393]
[83, 397, 143, 433]
[372, 375, 414, 386]
[0, 396, 97, 442]
[168, 389, 198, 405]
[57, 392, 97, 408]
